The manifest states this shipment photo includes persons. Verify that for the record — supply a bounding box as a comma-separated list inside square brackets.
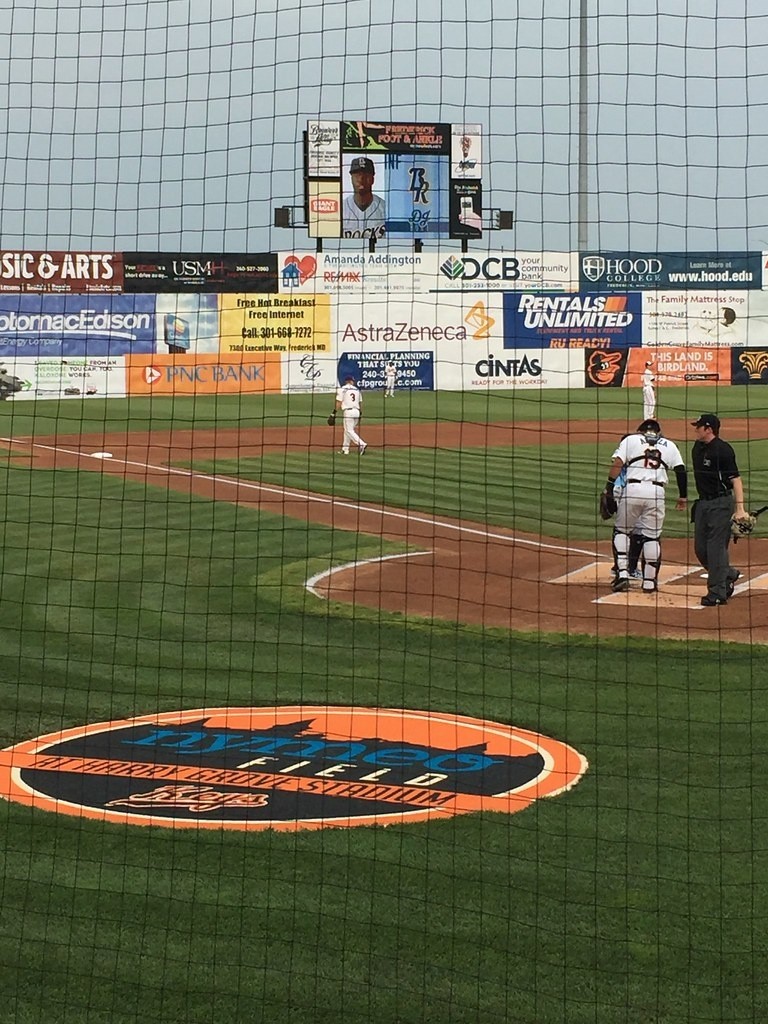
[690, 414, 751, 606]
[328, 377, 368, 455]
[342, 157, 386, 240]
[600, 419, 687, 593]
[641, 361, 657, 420]
[385, 361, 396, 398]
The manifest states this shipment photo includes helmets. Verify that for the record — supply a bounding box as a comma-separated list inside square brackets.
[638, 419, 661, 432]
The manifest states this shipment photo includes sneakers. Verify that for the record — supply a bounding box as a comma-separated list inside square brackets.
[610, 568, 619, 577]
[727, 569, 740, 597]
[337, 450, 350, 454]
[701, 591, 727, 606]
[628, 570, 642, 580]
[359, 442, 367, 455]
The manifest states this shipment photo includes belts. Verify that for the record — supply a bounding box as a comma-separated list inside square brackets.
[347, 408, 360, 410]
[628, 479, 663, 487]
[699, 490, 732, 500]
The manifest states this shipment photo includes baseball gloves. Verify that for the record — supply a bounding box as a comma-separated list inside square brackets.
[326, 412, 336, 426]
[730, 515, 756, 544]
[598, 491, 617, 521]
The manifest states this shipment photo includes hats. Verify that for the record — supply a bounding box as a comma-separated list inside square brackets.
[645, 360, 654, 366]
[389, 360, 392, 362]
[691, 413, 720, 428]
[345, 375, 355, 380]
[349, 157, 375, 176]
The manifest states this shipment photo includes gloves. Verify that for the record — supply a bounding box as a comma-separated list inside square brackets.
[328, 414, 335, 425]
[359, 412, 362, 419]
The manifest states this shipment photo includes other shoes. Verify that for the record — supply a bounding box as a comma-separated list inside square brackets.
[641, 589, 656, 593]
[612, 578, 630, 591]
[390, 395, 394, 397]
[384, 394, 388, 398]
